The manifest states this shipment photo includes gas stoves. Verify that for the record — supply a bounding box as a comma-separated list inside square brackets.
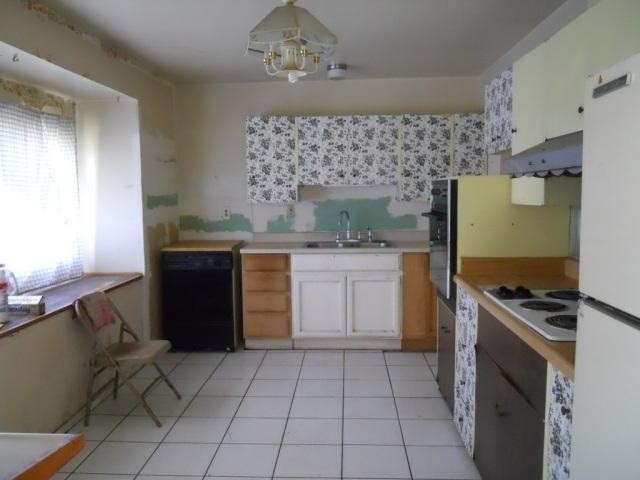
[484, 284, 576, 343]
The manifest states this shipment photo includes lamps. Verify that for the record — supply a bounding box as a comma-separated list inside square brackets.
[245, 0, 338, 82]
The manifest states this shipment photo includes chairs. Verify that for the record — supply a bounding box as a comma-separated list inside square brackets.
[75, 290, 183, 427]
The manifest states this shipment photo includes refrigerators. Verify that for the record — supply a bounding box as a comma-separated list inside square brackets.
[565, 52, 640, 480]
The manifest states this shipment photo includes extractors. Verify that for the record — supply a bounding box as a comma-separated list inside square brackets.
[500, 130, 582, 181]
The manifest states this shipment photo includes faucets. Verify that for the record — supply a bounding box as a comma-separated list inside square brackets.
[366, 225, 372, 241]
[338, 209, 351, 240]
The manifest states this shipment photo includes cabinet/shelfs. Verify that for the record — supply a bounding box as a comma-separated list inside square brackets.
[242, 253, 291, 340]
[545, 365, 575, 479]
[453, 282, 477, 460]
[245, 112, 488, 203]
[511, 0, 639, 157]
[404, 254, 437, 339]
[484, 67, 511, 155]
[291, 272, 402, 341]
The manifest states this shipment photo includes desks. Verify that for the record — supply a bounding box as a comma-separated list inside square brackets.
[0, 433, 85, 480]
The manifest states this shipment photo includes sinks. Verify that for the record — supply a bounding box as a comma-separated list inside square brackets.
[304, 240, 393, 249]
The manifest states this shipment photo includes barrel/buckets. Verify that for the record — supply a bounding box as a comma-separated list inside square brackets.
[0, 263, 19, 324]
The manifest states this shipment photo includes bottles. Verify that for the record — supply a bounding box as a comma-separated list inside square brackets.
[0, 264, 9, 324]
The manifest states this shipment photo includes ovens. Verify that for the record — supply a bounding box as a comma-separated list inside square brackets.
[418, 177, 457, 296]
